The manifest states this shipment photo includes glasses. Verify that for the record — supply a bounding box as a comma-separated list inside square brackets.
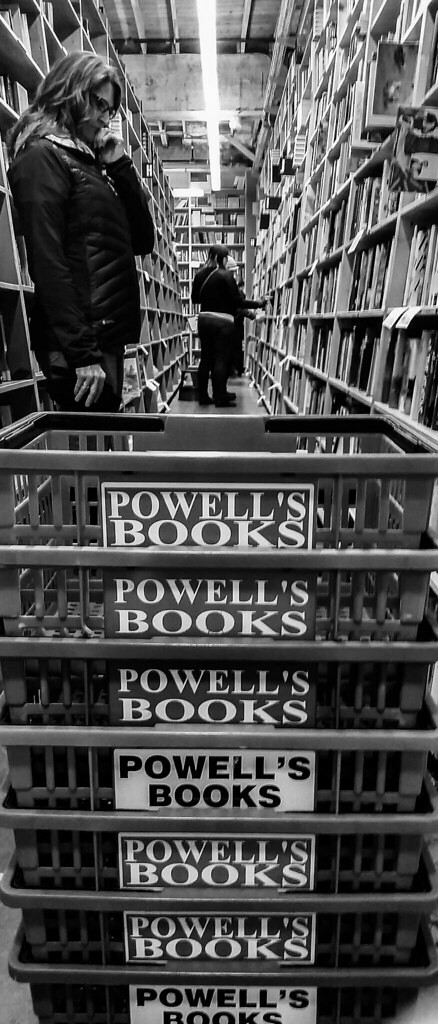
[88, 92, 117, 120]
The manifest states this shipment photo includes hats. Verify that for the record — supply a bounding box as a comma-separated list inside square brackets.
[225, 255, 239, 271]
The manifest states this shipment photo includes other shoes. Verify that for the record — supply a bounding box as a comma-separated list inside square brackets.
[214, 399, 237, 408]
[237, 367, 245, 377]
[212, 391, 236, 401]
[199, 397, 214, 406]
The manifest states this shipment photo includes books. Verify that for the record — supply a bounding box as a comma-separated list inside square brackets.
[0, 1, 438, 509]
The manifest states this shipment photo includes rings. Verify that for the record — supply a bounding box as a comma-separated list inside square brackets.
[84, 382, 90, 389]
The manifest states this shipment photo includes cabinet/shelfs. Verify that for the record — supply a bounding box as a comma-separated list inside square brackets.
[241, 0, 438, 547]
[173, 206, 245, 369]
[0, 0, 184, 425]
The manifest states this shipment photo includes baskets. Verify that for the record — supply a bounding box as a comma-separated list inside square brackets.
[0, 411, 438, 1023]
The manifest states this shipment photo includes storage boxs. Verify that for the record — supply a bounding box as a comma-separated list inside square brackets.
[0, 410, 438, 1024]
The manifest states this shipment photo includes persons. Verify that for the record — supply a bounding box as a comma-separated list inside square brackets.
[5, 50, 154, 525]
[192, 246, 274, 407]
